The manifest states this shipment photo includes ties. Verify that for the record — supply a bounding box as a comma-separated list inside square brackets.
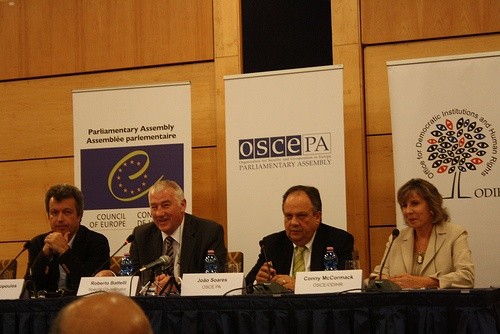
[293, 245, 305, 277]
[46, 259, 60, 293]
[162, 236, 173, 276]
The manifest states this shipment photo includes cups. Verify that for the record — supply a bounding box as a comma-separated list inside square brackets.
[224, 263, 238, 273]
[345, 260, 360, 270]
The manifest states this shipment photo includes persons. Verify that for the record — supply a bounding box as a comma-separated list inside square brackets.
[29, 184, 110, 292]
[94, 180, 227, 294]
[52, 291, 153, 334]
[365, 178, 475, 290]
[245, 186, 355, 292]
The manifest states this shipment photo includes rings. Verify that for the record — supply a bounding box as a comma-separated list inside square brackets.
[283, 279, 285, 283]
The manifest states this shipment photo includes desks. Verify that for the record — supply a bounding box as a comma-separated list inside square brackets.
[0, 285, 500, 334]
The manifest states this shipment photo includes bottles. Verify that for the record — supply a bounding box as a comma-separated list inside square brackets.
[204, 250, 218, 273]
[120, 253, 134, 276]
[323, 247, 338, 271]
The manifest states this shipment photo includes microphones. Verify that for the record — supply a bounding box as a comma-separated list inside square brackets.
[139, 255, 170, 273]
[0, 242, 32, 278]
[255, 240, 290, 293]
[374, 229, 401, 292]
[92, 234, 136, 276]
